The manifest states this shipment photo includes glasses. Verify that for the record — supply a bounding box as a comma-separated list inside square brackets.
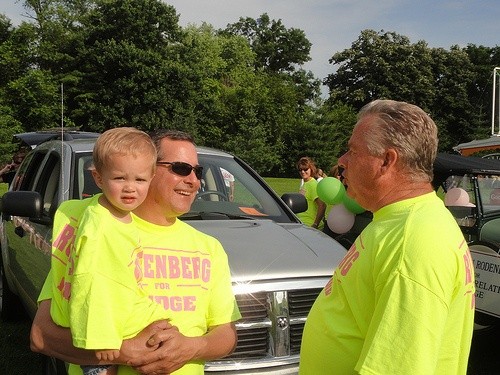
[157, 161, 204, 180]
[298, 167, 311, 171]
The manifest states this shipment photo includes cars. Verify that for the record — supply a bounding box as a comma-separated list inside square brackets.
[339, 153, 500, 335]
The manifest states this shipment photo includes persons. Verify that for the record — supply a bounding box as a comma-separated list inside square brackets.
[295, 156, 341, 230]
[0, 150, 97, 185]
[298, 99, 476, 375]
[219, 166, 235, 201]
[30, 130, 242, 375]
[69, 126, 173, 375]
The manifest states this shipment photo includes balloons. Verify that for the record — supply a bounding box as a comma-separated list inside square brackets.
[316, 177, 367, 233]
[444, 188, 476, 208]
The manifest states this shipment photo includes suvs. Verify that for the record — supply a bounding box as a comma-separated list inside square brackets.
[0, 129, 350, 375]
